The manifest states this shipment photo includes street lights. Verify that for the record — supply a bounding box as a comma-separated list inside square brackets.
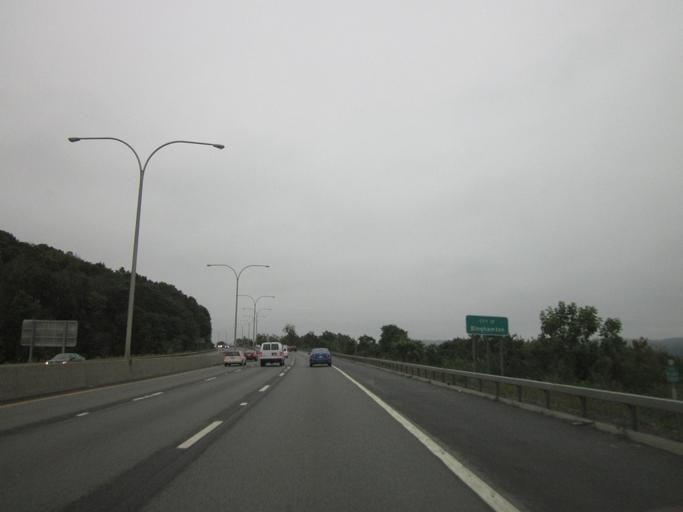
[69, 137, 226, 361]
[207, 263, 276, 352]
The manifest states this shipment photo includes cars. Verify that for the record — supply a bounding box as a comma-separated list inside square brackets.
[45, 353, 87, 366]
[310, 348, 331, 367]
[224, 342, 289, 367]
